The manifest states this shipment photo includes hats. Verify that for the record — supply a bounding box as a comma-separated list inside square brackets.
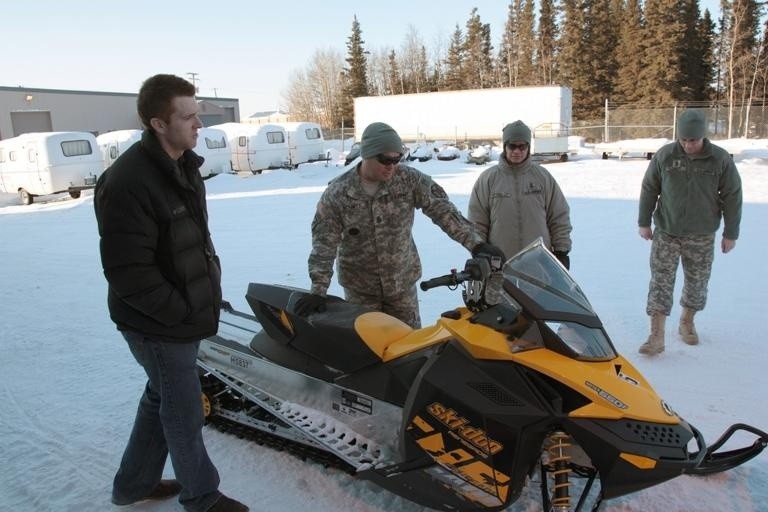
[360, 121, 405, 162]
[676, 108, 706, 139]
[502, 119, 531, 148]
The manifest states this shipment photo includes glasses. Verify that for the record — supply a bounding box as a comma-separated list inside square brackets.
[377, 153, 401, 166]
[505, 142, 529, 150]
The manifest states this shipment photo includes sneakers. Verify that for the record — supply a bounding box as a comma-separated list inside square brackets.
[184, 488, 250, 512]
[110, 477, 182, 505]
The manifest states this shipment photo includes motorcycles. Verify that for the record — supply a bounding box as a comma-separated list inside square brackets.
[194, 237, 768, 510]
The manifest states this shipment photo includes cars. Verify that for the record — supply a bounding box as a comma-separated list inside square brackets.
[344, 143, 361, 165]
[399, 144, 409, 158]
[436, 147, 461, 160]
[409, 148, 432, 162]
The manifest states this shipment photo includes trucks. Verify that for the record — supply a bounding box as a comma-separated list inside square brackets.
[530, 122, 570, 162]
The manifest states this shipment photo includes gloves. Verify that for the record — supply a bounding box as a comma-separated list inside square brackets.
[552, 250, 570, 272]
[471, 241, 506, 269]
[293, 291, 327, 316]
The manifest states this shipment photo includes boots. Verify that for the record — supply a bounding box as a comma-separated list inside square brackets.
[638, 312, 666, 355]
[679, 306, 700, 345]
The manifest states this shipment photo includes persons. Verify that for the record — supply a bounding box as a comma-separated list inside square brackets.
[637, 107, 743, 357]
[468, 121, 573, 273]
[94, 73, 252, 512]
[293, 122, 506, 328]
